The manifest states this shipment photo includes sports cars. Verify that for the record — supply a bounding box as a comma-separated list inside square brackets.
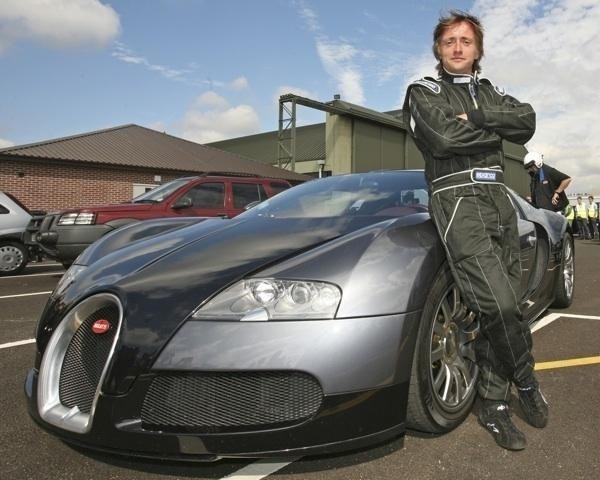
[26, 168, 575, 464]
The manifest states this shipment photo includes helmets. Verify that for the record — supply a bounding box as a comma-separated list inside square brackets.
[523, 152, 543, 168]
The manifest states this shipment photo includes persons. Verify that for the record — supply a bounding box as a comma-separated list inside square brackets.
[586, 196, 599, 239]
[575, 197, 592, 240]
[524, 160, 572, 215]
[564, 203, 575, 234]
[402, 8, 549, 451]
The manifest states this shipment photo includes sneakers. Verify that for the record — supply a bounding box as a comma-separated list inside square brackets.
[516, 380, 549, 428]
[478, 399, 526, 450]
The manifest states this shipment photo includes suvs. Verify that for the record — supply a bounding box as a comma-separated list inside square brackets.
[0, 187, 38, 276]
[20, 170, 304, 272]
